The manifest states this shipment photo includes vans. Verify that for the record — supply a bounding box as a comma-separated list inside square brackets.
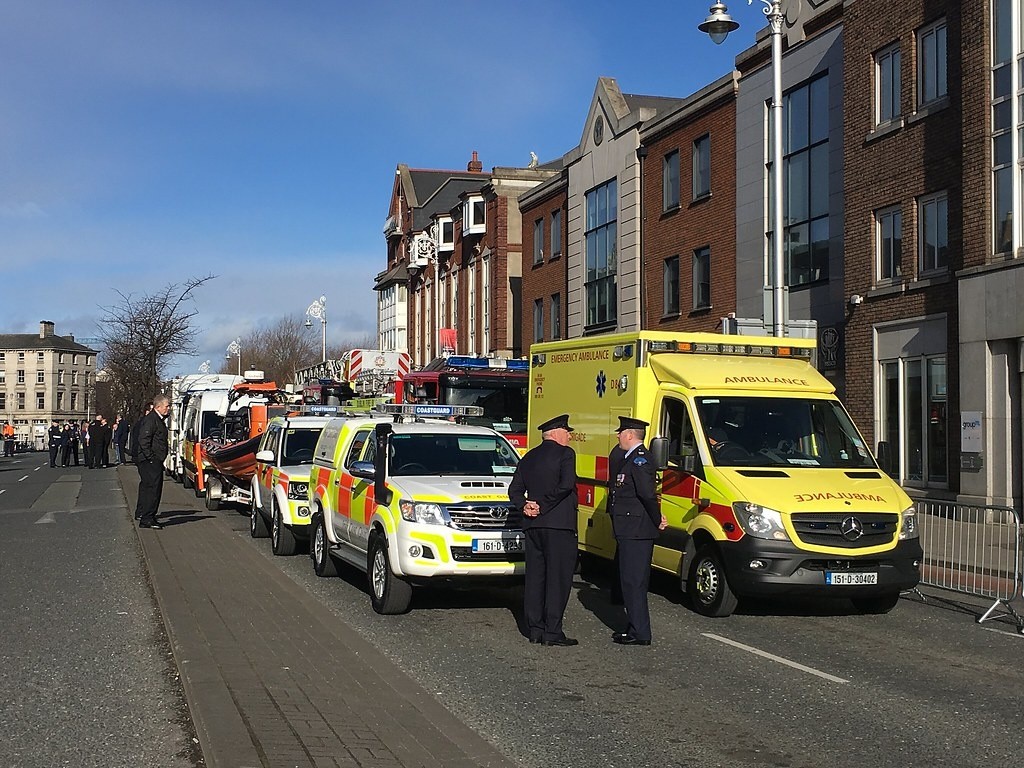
[182, 388, 231, 498]
[176, 378, 239, 481]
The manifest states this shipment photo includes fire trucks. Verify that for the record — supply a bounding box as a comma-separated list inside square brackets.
[401, 352, 529, 458]
[285, 348, 414, 414]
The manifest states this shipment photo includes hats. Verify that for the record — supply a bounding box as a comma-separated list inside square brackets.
[537, 414, 574, 432]
[68, 419, 75, 424]
[615, 416, 650, 432]
[52, 419, 59, 423]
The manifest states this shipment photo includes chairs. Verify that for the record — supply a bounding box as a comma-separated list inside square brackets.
[708, 428, 728, 445]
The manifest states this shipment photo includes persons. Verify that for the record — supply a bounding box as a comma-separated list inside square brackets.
[508, 414, 581, 646]
[131, 402, 153, 520]
[605, 416, 667, 644]
[3, 422, 14, 457]
[138, 394, 170, 530]
[48, 414, 128, 469]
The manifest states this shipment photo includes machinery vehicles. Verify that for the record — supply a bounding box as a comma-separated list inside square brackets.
[201, 364, 305, 512]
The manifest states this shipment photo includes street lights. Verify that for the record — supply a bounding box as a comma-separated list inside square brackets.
[699, 1, 785, 338]
[198, 360, 211, 375]
[303, 293, 326, 363]
[406, 223, 439, 359]
[225, 338, 241, 375]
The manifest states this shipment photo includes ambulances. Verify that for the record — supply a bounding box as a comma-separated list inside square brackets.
[528, 331, 925, 619]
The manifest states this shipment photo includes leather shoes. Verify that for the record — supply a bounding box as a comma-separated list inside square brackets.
[612, 629, 633, 638]
[135, 513, 143, 520]
[614, 635, 651, 645]
[529, 636, 541, 644]
[140, 521, 165, 529]
[541, 635, 578, 646]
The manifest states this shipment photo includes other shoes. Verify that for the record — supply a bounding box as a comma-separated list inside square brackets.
[50, 462, 111, 469]
[4, 455, 8, 457]
[11, 454, 14, 457]
[115, 459, 126, 465]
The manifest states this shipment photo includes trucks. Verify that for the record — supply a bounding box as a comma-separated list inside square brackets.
[162, 364, 244, 476]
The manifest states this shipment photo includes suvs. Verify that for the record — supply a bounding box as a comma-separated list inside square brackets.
[249, 404, 347, 556]
[308, 402, 527, 615]
